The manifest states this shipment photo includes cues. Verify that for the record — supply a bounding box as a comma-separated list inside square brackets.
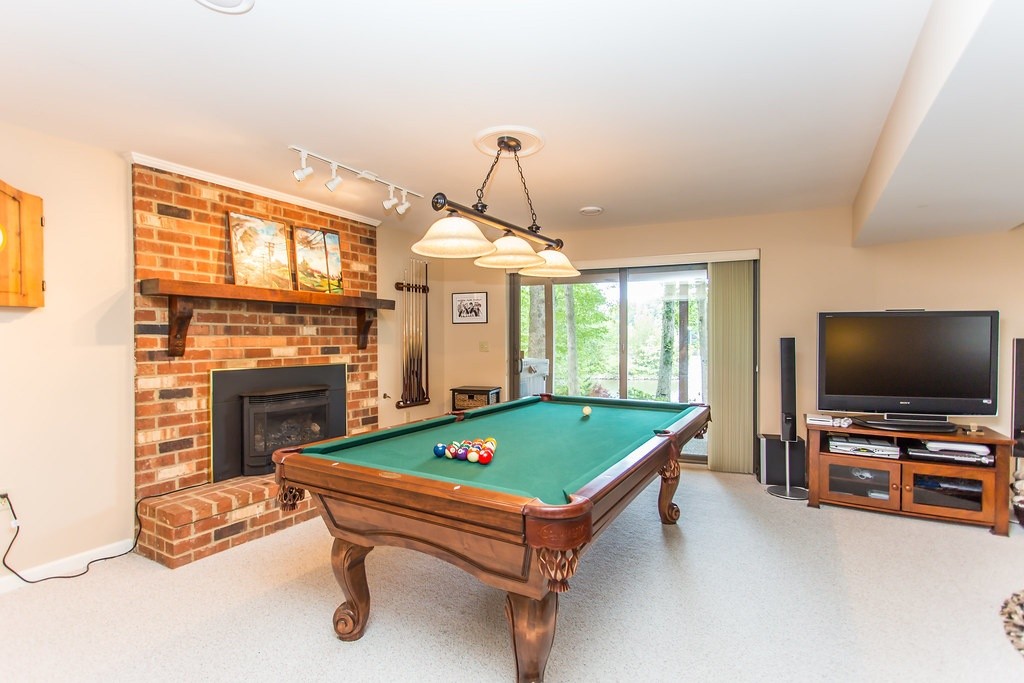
[402, 256, 430, 404]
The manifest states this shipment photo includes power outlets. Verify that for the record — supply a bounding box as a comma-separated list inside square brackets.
[0, 491, 11, 511]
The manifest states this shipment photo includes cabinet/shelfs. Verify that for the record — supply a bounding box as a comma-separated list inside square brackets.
[803, 413, 1018, 536]
[0, 180, 46, 307]
[449, 386, 501, 410]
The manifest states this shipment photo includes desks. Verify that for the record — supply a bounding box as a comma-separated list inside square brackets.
[273, 394, 713, 683]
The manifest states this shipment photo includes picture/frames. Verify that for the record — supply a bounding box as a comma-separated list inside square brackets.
[228, 211, 294, 290]
[321, 228, 345, 296]
[452, 292, 488, 324]
[292, 225, 331, 293]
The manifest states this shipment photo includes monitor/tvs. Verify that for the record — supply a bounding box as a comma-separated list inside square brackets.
[817, 311, 999, 425]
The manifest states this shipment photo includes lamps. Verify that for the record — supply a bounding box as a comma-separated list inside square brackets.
[383, 185, 398, 210]
[411, 137, 581, 278]
[325, 163, 343, 192]
[395, 190, 411, 215]
[293, 152, 314, 183]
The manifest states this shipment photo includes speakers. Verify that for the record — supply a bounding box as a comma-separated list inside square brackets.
[780, 337, 796, 442]
[1012, 338, 1024, 458]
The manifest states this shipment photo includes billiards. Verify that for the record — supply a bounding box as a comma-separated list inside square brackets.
[582, 406, 592, 415]
[433, 437, 497, 464]
